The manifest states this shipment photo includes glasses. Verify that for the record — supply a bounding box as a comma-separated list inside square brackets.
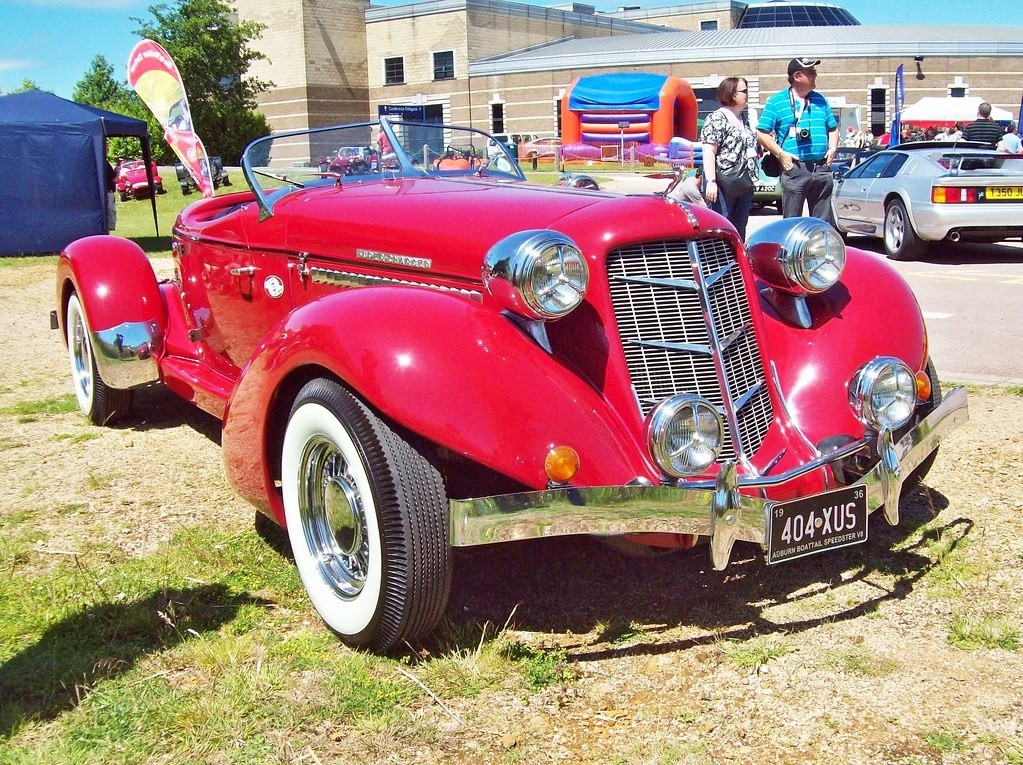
[799, 69, 816, 74]
[737, 89, 748, 95]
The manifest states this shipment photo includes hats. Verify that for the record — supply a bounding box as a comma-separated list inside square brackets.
[787, 58, 820, 75]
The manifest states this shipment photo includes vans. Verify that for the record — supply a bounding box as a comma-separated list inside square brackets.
[483, 133, 539, 160]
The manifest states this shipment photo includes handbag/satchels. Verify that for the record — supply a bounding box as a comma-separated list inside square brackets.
[761, 154, 783, 177]
[715, 144, 755, 202]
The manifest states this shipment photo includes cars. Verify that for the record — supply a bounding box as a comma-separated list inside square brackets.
[518, 138, 561, 162]
[830, 140, 1023, 261]
[54, 114, 966, 657]
[174, 156, 230, 195]
[113, 158, 163, 202]
[318, 146, 382, 179]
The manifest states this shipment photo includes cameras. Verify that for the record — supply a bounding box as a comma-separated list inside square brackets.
[796, 129, 812, 142]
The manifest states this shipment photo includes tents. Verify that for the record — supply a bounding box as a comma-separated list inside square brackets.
[0, 88, 160, 255]
[892, 96, 1012, 128]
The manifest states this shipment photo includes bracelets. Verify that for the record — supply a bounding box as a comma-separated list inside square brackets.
[706, 179, 716, 183]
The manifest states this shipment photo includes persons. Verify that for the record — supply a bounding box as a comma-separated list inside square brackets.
[699, 76, 760, 243]
[116, 334, 123, 360]
[1003, 125, 1023, 153]
[106, 143, 121, 235]
[845, 127, 874, 148]
[924, 121, 964, 141]
[754, 56, 838, 224]
[376, 125, 393, 172]
[670, 165, 707, 208]
[960, 102, 1004, 150]
